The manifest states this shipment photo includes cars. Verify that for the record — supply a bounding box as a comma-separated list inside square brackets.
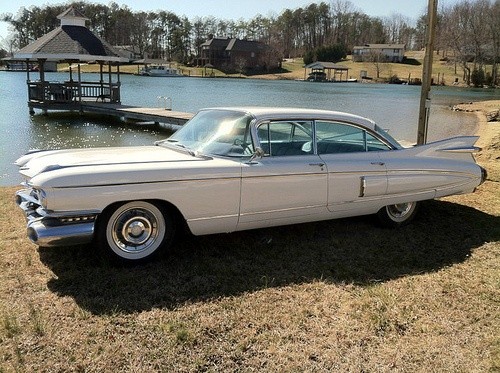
[13, 106, 487, 261]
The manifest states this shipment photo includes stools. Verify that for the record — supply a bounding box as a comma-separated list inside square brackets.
[97, 94, 111, 103]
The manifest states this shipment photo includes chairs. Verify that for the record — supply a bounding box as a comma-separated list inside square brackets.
[264, 137, 370, 162]
